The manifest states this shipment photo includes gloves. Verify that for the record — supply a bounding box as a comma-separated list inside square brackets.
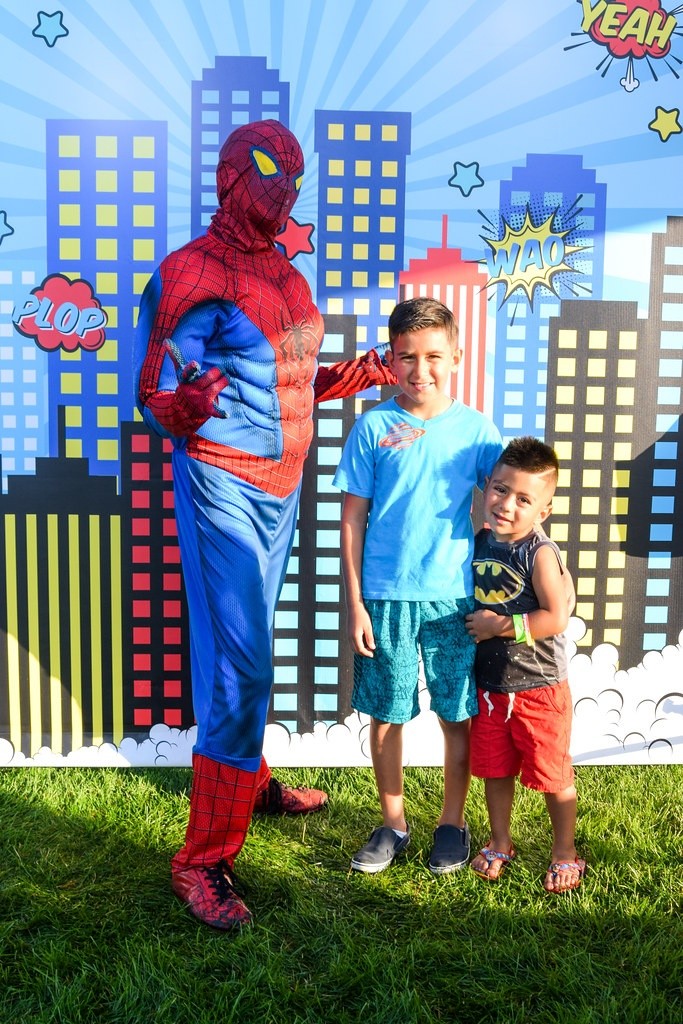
[162, 337, 230, 422]
[365, 342, 400, 387]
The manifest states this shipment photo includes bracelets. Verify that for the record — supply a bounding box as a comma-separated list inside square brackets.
[511, 614, 526, 644]
[523, 612, 534, 646]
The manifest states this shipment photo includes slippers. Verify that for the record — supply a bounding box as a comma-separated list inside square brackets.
[543, 855, 586, 893]
[470, 840, 516, 880]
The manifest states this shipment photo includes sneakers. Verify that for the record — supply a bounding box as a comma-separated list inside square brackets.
[253, 777, 328, 816]
[170, 858, 255, 930]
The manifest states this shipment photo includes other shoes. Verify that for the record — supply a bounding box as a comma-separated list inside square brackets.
[428, 820, 472, 875]
[349, 820, 412, 872]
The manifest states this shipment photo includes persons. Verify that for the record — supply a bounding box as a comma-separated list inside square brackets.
[136, 122, 399, 932]
[464, 437, 586, 893]
[332, 297, 506, 875]
